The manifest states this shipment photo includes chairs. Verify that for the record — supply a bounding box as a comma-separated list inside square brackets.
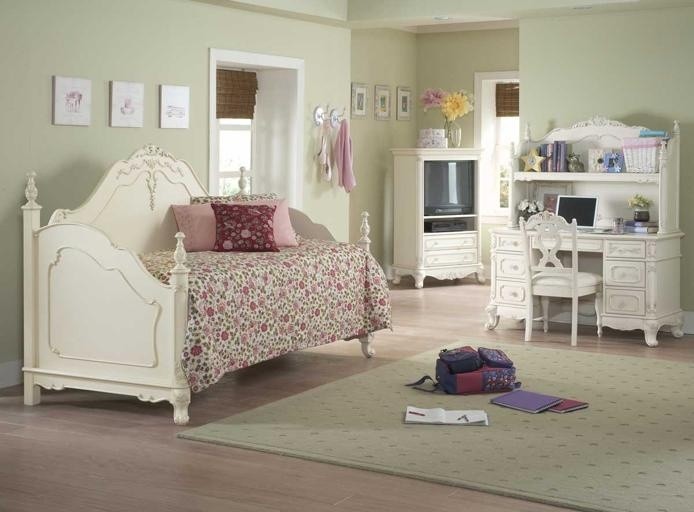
[518, 209, 603, 348]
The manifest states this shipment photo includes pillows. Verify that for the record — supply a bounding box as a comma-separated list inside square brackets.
[210, 201, 281, 253]
[170, 199, 300, 253]
[190, 192, 280, 205]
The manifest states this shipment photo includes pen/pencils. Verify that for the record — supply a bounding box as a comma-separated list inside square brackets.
[458, 415, 469, 421]
[409, 411, 425, 416]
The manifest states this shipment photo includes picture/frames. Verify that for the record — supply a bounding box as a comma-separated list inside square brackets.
[374, 84, 391, 121]
[396, 87, 413, 121]
[351, 81, 369, 117]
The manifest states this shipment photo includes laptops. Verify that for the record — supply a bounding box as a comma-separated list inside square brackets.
[543, 195, 599, 232]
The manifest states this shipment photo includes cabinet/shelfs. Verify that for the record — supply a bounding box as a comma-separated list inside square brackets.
[484, 227, 549, 331]
[507, 117, 682, 234]
[600, 231, 686, 349]
[389, 144, 487, 289]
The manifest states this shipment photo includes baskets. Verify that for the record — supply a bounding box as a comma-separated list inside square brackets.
[620, 134, 664, 175]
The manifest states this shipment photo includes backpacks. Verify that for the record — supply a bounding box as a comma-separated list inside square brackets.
[434, 345, 522, 396]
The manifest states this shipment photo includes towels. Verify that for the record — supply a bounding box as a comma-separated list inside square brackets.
[333, 118, 357, 194]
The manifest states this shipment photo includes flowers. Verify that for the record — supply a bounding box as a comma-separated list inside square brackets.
[517, 199, 545, 214]
[415, 86, 474, 122]
[627, 192, 655, 208]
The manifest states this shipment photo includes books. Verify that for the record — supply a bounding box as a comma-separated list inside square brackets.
[405, 405, 488, 426]
[490, 390, 589, 415]
[536, 140, 568, 171]
[624, 220, 657, 233]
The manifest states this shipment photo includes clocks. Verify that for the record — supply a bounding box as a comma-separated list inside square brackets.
[519, 148, 546, 172]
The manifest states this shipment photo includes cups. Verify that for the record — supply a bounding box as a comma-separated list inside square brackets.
[612, 217, 624, 235]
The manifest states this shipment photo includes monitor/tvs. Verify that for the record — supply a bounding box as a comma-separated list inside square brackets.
[424, 161, 474, 215]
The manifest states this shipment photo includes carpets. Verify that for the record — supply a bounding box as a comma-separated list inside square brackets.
[176, 337, 694, 511]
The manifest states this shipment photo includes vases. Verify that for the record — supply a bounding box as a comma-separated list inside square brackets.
[633, 207, 650, 222]
[440, 116, 462, 148]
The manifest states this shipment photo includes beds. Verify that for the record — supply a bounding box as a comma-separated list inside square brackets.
[19, 143, 394, 426]
